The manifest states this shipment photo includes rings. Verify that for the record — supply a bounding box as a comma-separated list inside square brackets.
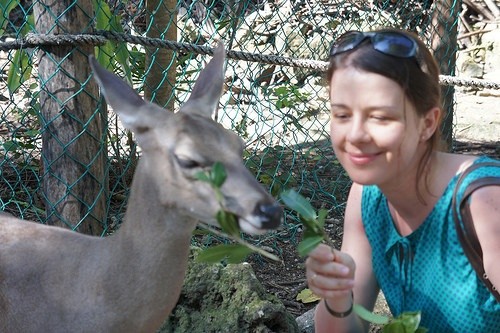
[310, 271, 318, 287]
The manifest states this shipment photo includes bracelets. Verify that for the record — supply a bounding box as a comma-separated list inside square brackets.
[322, 287, 354, 318]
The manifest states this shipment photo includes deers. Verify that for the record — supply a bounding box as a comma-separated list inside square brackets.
[0, 40, 283, 333]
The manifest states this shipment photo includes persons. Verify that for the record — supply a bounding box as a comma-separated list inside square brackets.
[305, 26, 500, 333]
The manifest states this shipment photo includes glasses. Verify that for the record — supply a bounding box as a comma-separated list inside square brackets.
[329, 29, 429, 74]
